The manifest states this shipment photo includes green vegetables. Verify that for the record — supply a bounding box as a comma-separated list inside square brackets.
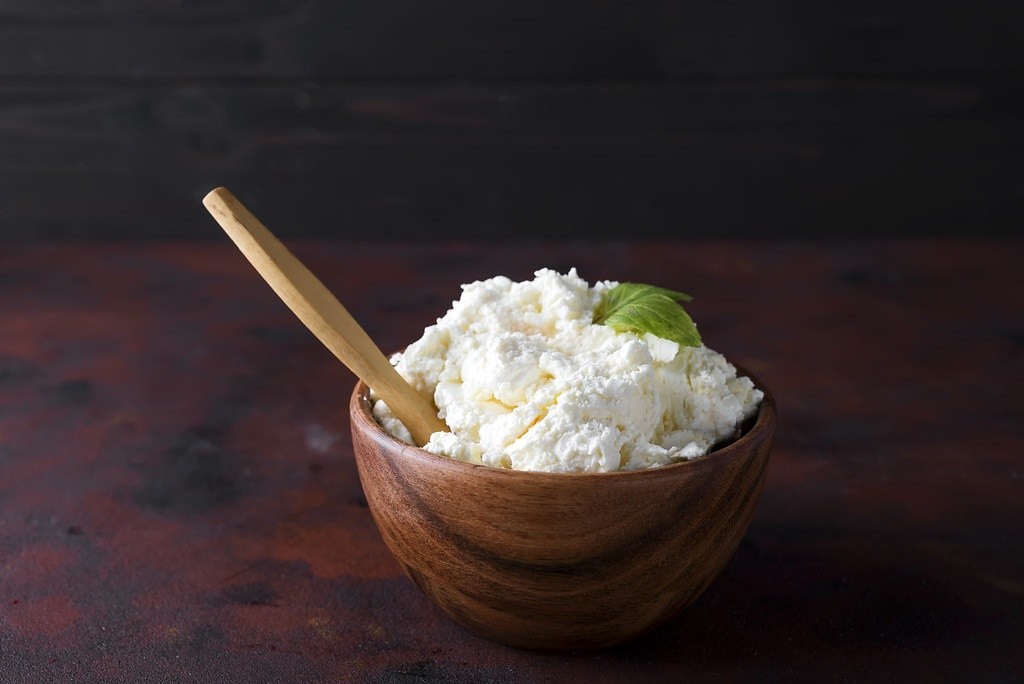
[591, 283, 703, 348]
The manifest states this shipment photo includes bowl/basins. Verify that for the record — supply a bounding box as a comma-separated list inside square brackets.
[349, 350, 778, 649]
